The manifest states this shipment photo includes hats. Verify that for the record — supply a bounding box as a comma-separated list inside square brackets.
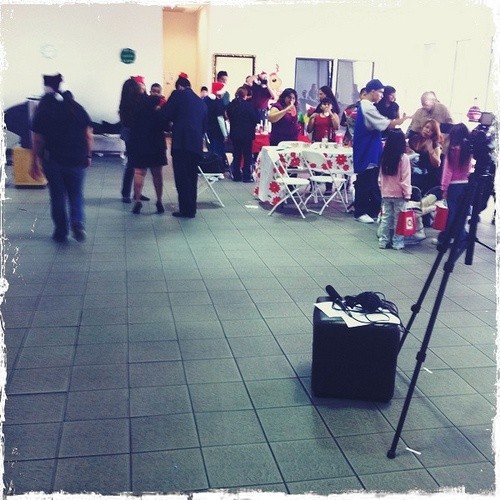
[366, 79, 387, 93]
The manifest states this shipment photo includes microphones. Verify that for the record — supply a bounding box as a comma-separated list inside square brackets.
[325, 284, 353, 318]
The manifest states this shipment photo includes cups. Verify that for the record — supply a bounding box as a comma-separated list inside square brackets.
[321, 138, 328, 149]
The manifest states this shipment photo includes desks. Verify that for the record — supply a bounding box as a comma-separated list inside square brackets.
[254, 145, 354, 204]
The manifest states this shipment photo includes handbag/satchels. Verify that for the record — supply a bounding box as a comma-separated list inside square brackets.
[396, 202, 416, 237]
[433, 199, 448, 231]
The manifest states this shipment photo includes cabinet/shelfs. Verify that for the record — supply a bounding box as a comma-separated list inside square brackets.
[13, 145, 47, 189]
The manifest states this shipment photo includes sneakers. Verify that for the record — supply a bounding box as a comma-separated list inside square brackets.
[353, 214, 375, 223]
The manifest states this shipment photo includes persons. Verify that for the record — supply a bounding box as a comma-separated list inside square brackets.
[340, 80, 500, 250]
[29, 74, 93, 242]
[201, 71, 301, 195]
[118, 72, 207, 218]
[307, 86, 340, 195]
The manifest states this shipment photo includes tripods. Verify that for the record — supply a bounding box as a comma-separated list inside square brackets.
[385, 152, 496, 460]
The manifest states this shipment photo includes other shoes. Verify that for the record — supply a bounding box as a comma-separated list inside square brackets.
[431, 237, 439, 245]
[132, 201, 142, 213]
[378, 240, 389, 249]
[392, 243, 406, 249]
[52, 229, 88, 243]
[233, 176, 254, 182]
[156, 202, 164, 212]
[122, 198, 131, 203]
[172, 212, 196, 218]
[141, 195, 150, 201]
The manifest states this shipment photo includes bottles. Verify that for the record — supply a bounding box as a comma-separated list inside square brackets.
[290, 102, 296, 117]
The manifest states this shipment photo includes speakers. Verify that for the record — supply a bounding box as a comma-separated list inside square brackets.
[311, 296, 399, 402]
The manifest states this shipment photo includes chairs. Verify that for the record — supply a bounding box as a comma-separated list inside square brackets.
[267, 141, 359, 218]
[197, 166, 226, 207]
